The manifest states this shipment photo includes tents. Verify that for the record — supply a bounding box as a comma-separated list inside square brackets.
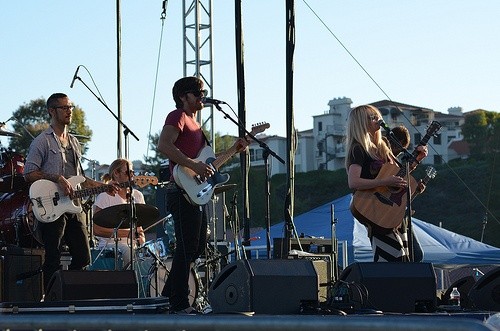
[230, 193, 500, 265]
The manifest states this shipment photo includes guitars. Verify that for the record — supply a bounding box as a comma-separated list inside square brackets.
[172, 121, 270, 207]
[348, 120, 442, 233]
[28, 170, 158, 224]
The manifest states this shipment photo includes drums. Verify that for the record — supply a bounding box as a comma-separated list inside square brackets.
[86, 247, 124, 271]
[0, 151, 26, 192]
[162, 214, 211, 252]
[135, 236, 176, 257]
[0, 189, 68, 249]
[124, 256, 202, 314]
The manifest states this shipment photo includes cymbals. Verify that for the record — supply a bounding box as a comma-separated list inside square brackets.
[212, 183, 238, 193]
[0, 129, 24, 138]
[92, 203, 160, 229]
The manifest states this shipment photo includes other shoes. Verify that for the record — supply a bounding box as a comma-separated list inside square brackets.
[175, 307, 202, 315]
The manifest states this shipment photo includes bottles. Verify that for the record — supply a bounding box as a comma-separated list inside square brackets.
[450, 288, 460, 306]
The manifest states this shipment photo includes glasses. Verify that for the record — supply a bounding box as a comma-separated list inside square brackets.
[117, 168, 134, 177]
[52, 106, 75, 112]
[367, 114, 380, 120]
[183, 89, 208, 98]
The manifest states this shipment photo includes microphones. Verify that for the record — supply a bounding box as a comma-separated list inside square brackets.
[70, 66, 80, 87]
[378, 119, 395, 135]
[16, 270, 40, 280]
[202, 97, 227, 104]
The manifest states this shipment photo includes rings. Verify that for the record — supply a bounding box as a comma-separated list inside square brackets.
[206, 167, 208, 170]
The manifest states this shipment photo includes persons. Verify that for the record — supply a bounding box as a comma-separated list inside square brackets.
[388, 126, 424, 263]
[24, 92, 121, 301]
[158, 76, 251, 314]
[93, 158, 159, 266]
[346, 105, 428, 263]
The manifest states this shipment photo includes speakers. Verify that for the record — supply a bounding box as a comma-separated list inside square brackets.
[331, 261, 436, 312]
[208, 258, 318, 313]
[44, 270, 138, 303]
[443, 267, 500, 312]
[0, 249, 44, 302]
